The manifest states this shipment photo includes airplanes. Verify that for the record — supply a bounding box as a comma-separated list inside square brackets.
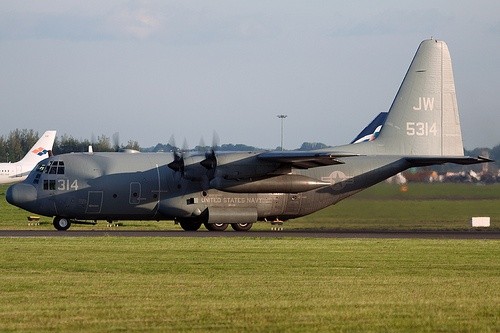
[7, 39, 494, 232]
[0, 130, 56, 185]
